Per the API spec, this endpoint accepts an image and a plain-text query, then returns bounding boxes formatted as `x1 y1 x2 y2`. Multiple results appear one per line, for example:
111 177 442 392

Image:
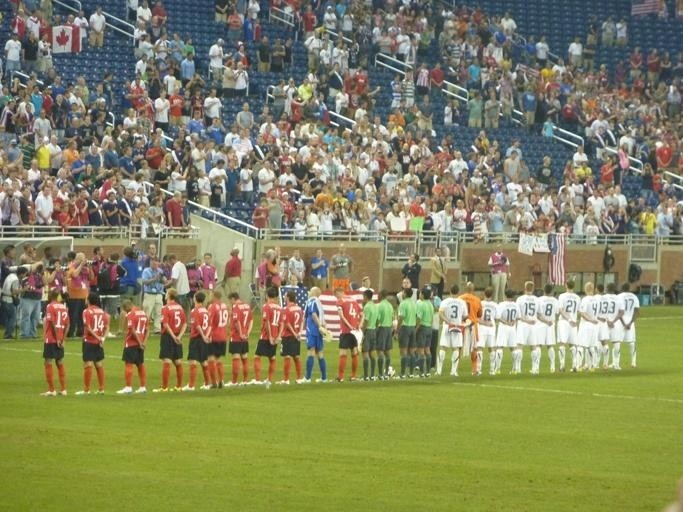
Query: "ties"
439 258 443 273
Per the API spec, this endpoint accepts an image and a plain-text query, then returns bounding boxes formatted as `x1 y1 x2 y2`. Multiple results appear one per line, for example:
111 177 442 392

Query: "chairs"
0 2 679 239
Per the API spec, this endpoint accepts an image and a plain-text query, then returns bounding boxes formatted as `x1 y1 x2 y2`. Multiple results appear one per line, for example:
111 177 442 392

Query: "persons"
0 1 683 340
330 281 640 382
40 287 110 397
115 286 328 395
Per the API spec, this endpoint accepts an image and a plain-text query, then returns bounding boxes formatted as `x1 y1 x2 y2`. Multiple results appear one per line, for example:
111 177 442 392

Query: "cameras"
46 265 56 271
60 265 69 272
195 281 202 287
160 272 166 276
84 260 97 266
185 262 196 269
273 256 288 264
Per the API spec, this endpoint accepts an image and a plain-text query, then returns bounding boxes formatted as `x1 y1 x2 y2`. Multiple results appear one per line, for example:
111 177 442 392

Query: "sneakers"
335 373 431 383
200 382 210 390
94 390 104 395
182 384 195 391
75 390 90 396
58 390 68 396
116 386 132 394
169 385 182 392
211 378 329 389
135 386 147 393
153 385 168 392
41 390 56 397
435 361 636 376
